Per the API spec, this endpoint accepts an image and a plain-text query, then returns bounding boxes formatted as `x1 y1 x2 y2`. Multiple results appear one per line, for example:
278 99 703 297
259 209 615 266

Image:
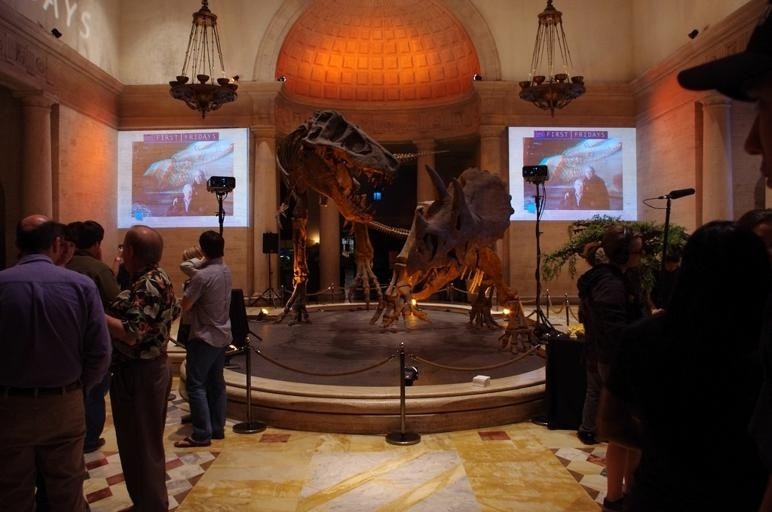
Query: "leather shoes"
175 438 211 447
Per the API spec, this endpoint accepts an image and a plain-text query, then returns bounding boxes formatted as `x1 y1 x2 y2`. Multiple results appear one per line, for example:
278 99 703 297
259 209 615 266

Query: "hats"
677 6 772 103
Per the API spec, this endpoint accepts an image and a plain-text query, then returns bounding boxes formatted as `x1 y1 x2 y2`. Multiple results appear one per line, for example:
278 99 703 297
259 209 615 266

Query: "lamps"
517 0 586 119
168 0 240 122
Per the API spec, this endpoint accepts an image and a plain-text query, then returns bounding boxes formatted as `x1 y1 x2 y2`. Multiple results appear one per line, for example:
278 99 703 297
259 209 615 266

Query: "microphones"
658 188 695 199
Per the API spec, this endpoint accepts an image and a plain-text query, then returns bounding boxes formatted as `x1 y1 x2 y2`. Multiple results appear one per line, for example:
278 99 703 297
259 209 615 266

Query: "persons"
561 178 596 210
572 207 771 510
165 183 209 216
673 5 771 196
0 210 182 512
564 165 611 210
172 229 235 448
177 242 208 426
171 168 222 216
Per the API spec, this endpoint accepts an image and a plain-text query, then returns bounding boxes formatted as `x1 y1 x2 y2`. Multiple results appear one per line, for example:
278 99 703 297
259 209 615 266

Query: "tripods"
249 254 281 307
527 184 556 331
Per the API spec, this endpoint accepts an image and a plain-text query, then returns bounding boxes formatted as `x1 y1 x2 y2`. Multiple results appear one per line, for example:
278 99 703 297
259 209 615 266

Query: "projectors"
206 176 235 192
523 165 550 182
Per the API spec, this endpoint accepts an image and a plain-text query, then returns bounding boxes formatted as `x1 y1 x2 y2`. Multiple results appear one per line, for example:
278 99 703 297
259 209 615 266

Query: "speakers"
263 233 279 252
